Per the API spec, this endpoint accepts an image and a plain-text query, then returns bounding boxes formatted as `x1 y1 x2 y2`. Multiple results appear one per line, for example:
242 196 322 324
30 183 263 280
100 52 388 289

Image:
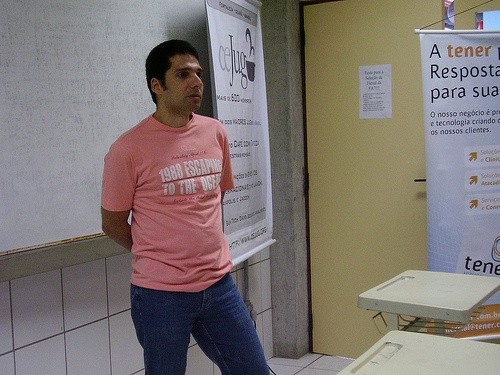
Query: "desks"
334 328 500 375
355 269 500 331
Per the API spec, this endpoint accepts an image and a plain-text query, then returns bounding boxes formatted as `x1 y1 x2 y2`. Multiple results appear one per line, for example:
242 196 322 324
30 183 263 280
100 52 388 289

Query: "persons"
100 40 269 375
